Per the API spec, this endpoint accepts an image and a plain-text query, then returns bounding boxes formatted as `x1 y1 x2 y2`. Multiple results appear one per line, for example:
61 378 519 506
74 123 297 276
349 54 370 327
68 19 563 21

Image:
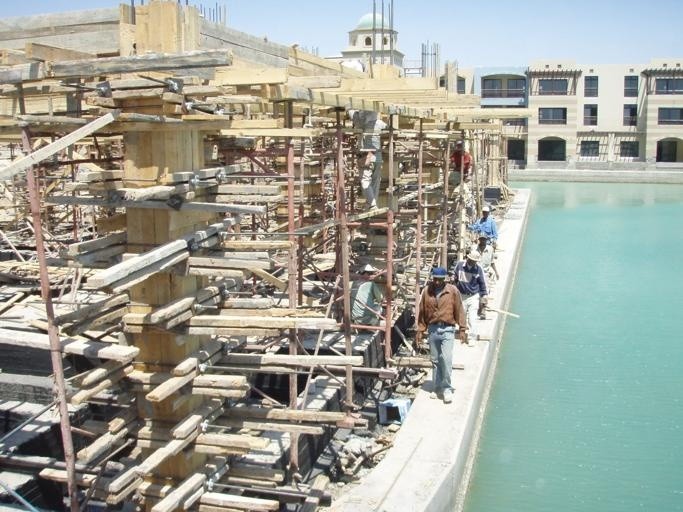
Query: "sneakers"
430 388 441 399
443 388 453 404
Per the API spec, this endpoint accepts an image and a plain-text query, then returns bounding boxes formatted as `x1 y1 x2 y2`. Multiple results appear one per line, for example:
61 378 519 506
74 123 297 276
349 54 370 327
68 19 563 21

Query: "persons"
447 138 471 185
465 205 497 257
449 250 489 347
469 234 500 303
415 266 469 403
351 265 383 332
345 109 388 211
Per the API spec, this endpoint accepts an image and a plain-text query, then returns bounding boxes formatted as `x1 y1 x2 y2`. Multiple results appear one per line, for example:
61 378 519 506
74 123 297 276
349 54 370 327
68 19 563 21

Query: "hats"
479 232 487 239
359 264 377 274
481 206 490 212
466 251 482 262
431 267 447 279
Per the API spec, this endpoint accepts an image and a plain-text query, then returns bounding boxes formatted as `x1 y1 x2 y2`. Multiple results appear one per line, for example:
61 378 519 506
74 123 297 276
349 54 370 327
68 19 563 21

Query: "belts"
432 322 453 327
